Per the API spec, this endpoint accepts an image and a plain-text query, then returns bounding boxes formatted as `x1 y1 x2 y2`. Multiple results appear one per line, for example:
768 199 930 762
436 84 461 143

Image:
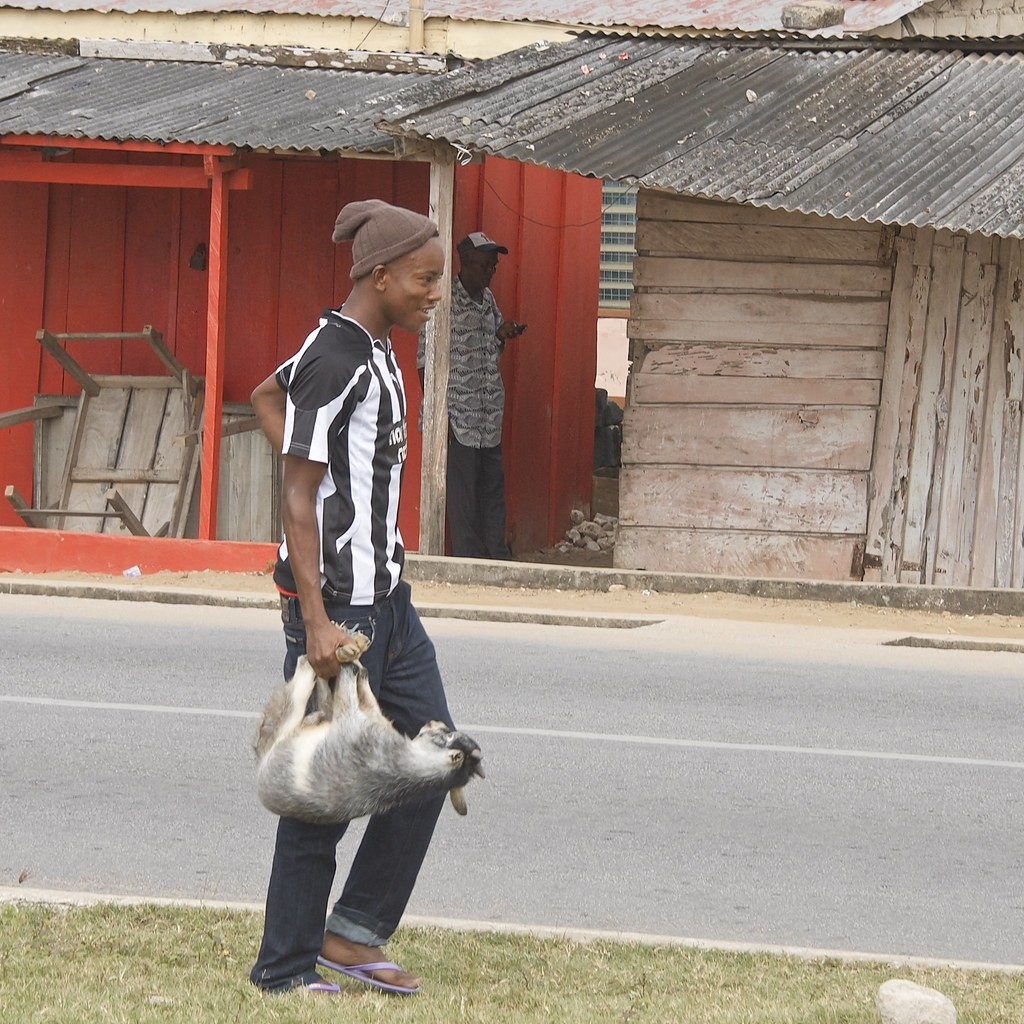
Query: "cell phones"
515 324 525 332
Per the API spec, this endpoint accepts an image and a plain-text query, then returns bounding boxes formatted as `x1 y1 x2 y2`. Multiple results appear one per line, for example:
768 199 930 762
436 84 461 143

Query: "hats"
331 199 438 280
458 231 510 254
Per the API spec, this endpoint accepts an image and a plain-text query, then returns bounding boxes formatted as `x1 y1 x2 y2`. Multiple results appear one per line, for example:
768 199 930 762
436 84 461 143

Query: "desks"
3 325 210 532
0 393 283 544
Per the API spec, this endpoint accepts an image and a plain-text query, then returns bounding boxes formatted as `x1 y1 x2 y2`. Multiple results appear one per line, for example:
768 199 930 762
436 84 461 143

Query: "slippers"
309 978 340 995
317 955 419 993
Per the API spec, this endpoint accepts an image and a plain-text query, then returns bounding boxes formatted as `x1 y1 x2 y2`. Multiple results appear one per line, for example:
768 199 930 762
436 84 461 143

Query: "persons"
416 231 525 563
250 200 455 996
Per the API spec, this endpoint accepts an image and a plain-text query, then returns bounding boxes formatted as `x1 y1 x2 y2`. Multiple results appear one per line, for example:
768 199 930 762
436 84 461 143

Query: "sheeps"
248 619 485 825
